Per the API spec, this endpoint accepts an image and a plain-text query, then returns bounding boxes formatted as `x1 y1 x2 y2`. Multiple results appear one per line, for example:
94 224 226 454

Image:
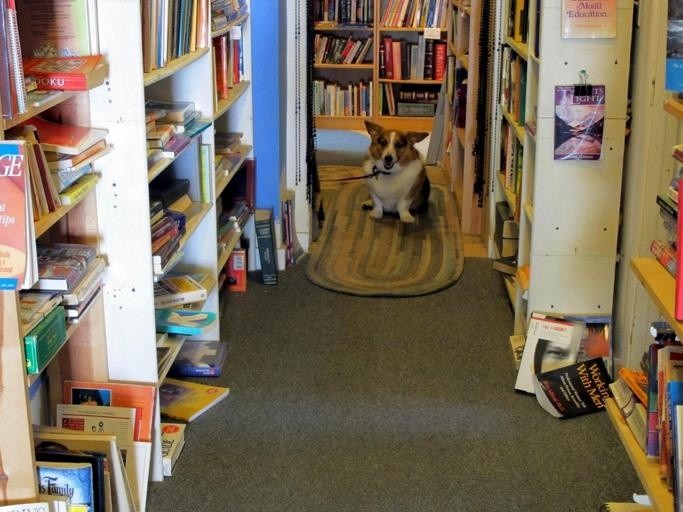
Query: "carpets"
302 178 465 297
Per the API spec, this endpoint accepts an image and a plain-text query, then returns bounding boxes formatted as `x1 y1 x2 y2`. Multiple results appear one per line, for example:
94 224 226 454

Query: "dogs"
361 120 430 224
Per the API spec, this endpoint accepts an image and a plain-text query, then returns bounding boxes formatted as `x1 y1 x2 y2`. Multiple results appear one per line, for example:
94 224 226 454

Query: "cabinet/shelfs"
312 0 376 131
377 1 449 130
0 0 113 512
87 0 217 482
603 2 682 512
488 0 634 374
440 1 483 235
210 1 252 333
